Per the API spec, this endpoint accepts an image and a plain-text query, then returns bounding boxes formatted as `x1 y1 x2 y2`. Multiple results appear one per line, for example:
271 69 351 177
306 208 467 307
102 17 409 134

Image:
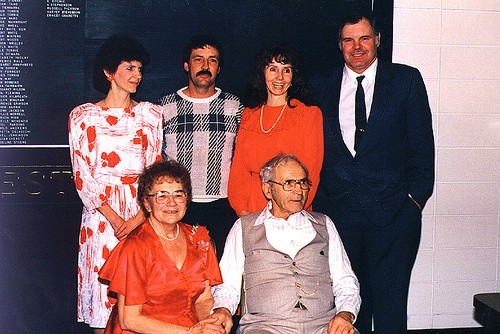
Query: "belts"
250 302 336 315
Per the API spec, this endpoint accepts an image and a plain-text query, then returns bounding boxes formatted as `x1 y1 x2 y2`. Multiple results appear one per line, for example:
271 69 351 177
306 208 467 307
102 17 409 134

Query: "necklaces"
148 217 180 241
260 100 287 133
104 99 132 122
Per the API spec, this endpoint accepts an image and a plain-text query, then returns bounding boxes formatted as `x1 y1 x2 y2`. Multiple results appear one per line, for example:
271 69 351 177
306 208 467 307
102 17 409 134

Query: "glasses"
267 178 312 191
145 190 189 204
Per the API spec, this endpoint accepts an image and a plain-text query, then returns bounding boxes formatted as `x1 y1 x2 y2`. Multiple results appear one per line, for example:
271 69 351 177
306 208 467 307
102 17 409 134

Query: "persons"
68 34 163 334
311 10 435 334
228 44 326 217
156 35 242 263
97 160 227 334
211 154 363 334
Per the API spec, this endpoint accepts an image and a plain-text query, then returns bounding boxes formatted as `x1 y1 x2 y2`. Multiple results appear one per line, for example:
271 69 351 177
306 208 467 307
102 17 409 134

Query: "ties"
354 75 368 152
